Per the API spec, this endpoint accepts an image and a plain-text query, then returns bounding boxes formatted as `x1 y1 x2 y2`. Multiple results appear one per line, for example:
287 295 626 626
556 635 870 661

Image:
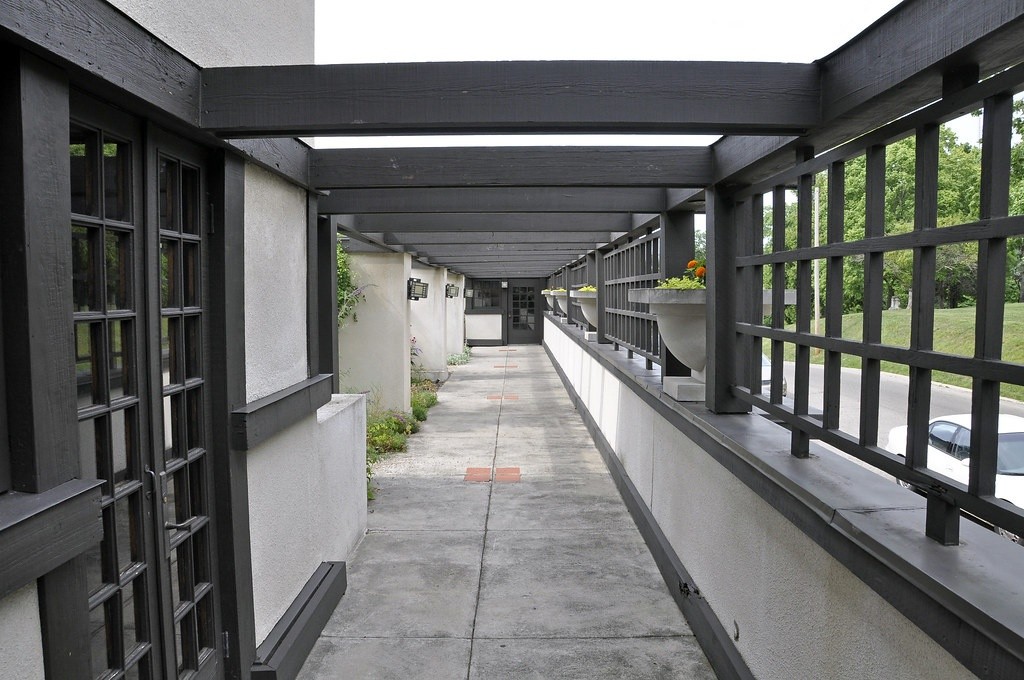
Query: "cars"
761 352 789 398
887 412 1024 544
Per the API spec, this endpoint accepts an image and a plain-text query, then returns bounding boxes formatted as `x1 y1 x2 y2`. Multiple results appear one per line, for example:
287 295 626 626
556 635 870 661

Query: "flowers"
656 259 706 290
578 285 596 292
552 287 566 291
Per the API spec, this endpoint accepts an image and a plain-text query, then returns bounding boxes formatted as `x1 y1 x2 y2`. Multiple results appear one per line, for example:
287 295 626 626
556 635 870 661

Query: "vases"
550 290 567 324
628 289 797 401
569 290 598 341
541 291 553 316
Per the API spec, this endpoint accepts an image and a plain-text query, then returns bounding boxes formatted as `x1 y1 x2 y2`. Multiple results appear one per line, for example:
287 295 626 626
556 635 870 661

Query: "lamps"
464 288 473 298
445 284 459 298
407 278 429 301
